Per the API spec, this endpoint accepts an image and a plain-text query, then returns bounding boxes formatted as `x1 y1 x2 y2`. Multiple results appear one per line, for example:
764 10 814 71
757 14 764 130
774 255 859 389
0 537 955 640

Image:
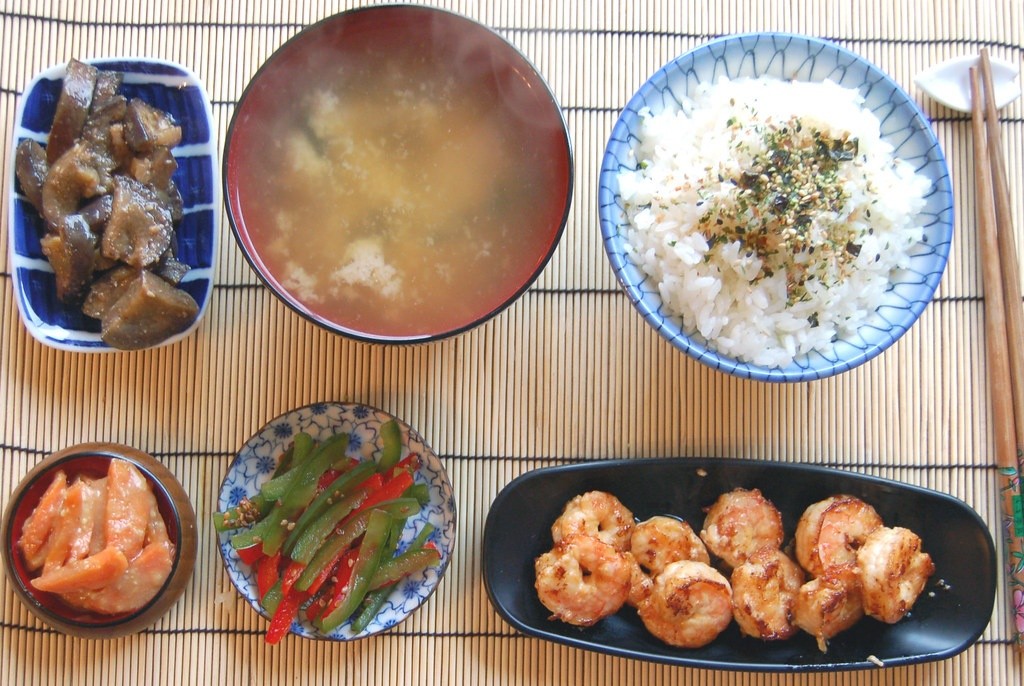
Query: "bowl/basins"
2 442 197 639
220 3 574 344
597 34 953 384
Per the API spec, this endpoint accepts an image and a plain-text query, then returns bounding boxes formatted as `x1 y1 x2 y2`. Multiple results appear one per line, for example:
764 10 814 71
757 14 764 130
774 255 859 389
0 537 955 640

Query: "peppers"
211 419 441 643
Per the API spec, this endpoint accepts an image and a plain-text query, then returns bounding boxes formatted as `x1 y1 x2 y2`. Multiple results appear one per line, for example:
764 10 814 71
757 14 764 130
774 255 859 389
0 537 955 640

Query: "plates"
483 464 996 670
216 402 457 640
12 57 221 351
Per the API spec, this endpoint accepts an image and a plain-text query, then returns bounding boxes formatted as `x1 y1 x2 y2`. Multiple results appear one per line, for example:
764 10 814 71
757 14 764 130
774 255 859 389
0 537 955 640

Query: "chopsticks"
970 47 1023 659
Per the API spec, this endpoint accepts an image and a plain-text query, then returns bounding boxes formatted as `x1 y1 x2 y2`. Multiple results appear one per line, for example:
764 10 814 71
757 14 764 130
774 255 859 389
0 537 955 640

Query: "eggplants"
17 58 200 353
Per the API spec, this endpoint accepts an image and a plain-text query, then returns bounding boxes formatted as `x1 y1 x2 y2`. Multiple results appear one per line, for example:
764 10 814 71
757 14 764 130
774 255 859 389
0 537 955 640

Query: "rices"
620 73 932 369
